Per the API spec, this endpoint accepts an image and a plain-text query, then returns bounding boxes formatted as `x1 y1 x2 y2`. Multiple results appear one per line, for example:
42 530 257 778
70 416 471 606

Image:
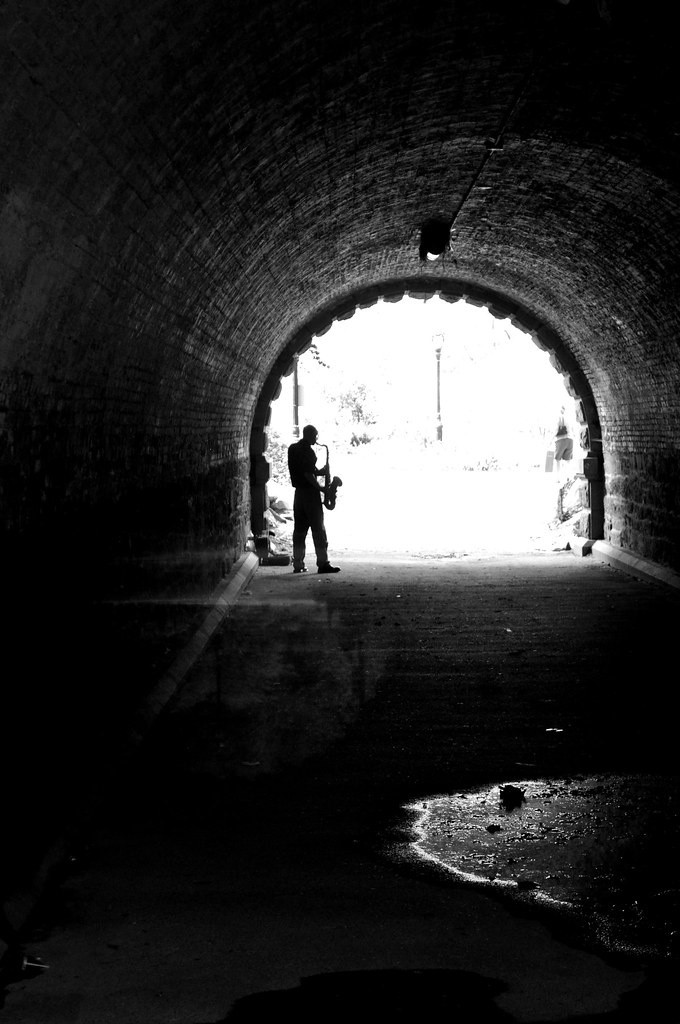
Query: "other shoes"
318 563 341 573
294 566 307 573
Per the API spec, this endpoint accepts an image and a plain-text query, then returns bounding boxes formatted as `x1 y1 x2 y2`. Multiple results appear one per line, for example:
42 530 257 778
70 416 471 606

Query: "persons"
287 425 343 574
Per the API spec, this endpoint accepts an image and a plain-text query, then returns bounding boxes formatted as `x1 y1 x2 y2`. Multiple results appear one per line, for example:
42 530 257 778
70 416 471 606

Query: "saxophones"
316 442 342 510
